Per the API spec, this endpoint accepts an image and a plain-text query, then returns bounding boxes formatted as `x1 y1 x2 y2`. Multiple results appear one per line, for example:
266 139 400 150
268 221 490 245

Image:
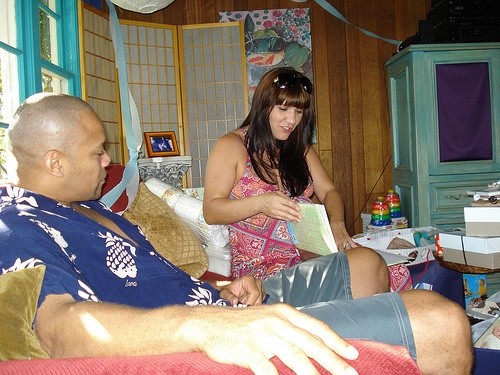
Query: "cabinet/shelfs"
383 42 500 297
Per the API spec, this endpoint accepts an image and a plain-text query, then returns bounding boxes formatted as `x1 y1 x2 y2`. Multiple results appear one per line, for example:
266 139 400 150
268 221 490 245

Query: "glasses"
272 73 312 94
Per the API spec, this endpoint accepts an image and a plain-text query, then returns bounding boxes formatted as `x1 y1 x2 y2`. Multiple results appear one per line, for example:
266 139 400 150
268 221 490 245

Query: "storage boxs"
463 206 500 237
205 243 232 277
353 226 466 311
439 231 500 269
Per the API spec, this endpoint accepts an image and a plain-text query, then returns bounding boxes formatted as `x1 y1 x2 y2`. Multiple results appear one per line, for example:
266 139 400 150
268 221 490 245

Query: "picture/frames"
144 131 181 158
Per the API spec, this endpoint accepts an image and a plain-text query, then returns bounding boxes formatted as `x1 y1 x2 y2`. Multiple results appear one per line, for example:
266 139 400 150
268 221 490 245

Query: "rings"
346 244 350 246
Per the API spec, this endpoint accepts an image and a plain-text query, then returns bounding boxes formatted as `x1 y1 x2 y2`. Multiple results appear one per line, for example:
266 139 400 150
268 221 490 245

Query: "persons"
150 136 172 152
478 279 487 300
203 68 412 294
0 92 473 375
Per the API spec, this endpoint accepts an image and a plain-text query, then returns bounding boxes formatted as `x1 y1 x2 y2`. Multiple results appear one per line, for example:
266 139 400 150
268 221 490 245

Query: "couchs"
0 162 425 375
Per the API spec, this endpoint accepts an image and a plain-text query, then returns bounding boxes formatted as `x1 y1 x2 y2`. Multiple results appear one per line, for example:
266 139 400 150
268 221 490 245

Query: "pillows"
123 181 209 278
0 264 51 362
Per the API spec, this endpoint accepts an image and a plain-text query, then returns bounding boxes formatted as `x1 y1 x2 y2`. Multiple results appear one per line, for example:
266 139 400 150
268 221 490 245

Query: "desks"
436 255 500 274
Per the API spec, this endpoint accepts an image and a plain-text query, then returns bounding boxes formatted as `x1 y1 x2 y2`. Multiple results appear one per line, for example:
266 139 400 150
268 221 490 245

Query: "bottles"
371 196 392 226
386 189 403 218
